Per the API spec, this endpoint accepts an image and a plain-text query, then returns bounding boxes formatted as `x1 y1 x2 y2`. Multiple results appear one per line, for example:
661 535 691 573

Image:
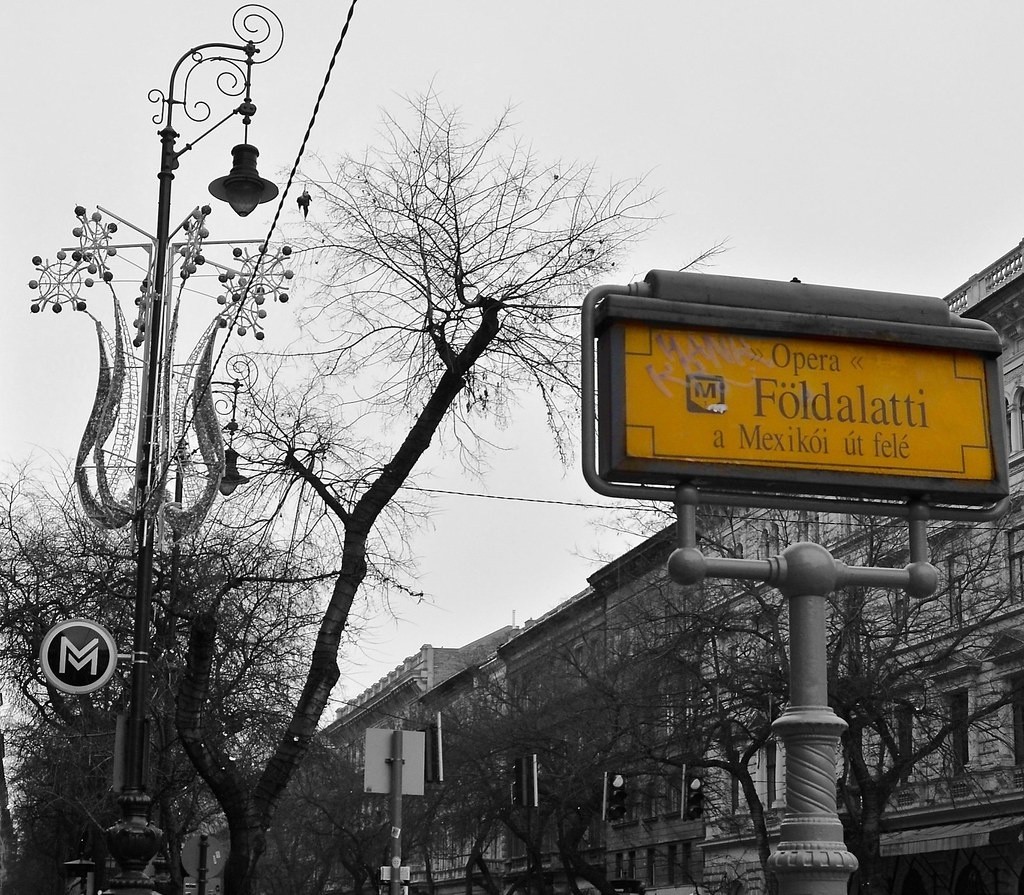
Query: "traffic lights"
607 772 628 820
686 775 705 820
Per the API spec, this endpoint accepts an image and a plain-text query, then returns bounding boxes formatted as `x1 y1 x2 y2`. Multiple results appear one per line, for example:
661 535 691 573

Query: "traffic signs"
582 270 1008 521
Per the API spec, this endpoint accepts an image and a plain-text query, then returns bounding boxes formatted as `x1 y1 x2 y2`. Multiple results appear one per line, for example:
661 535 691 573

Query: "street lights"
30 2 293 893
